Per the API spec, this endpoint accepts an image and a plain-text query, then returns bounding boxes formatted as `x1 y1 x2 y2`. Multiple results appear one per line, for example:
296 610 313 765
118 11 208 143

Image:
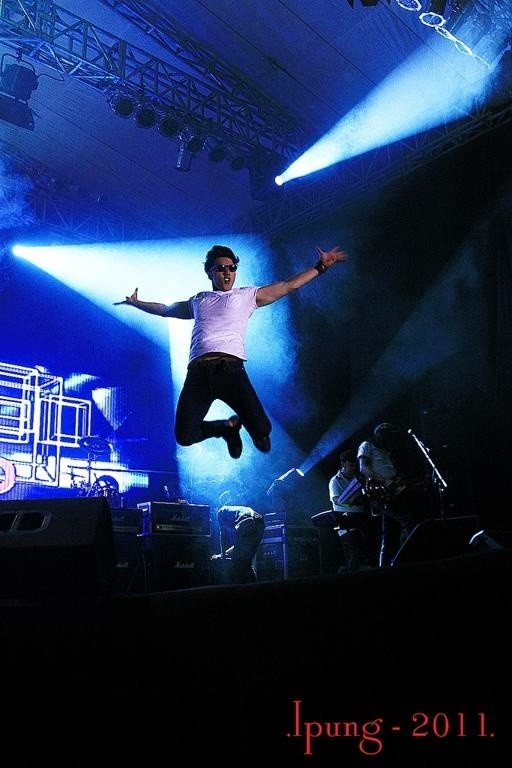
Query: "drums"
93 486 121 505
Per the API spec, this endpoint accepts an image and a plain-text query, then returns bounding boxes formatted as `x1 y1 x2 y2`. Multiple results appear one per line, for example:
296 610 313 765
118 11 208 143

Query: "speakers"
0 497 116 588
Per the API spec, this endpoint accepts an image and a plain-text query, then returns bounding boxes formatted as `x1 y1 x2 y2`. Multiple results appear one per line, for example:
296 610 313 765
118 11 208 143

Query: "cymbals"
77 435 113 457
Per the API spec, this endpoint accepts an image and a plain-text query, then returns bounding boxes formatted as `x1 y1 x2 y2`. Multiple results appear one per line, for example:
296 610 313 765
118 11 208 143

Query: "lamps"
179 120 205 156
174 145 196 175
205 135 230 164
0 47 66 133
156 114 179 141
132 103 160 131
253 177 285 204
108 90 136 119
225 145 248 175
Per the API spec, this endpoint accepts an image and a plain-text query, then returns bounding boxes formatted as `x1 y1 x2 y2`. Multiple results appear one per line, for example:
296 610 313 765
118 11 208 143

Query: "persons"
112 245 350 460
208 505 266 585
357 421 422 548
328 450 370 567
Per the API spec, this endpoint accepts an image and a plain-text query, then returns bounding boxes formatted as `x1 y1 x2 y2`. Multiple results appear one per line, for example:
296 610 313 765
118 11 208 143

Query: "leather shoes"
252 436 272 453
226 415 242 460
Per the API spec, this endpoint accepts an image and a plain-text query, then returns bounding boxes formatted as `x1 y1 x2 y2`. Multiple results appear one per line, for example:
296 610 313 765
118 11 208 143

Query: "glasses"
210 263 239 274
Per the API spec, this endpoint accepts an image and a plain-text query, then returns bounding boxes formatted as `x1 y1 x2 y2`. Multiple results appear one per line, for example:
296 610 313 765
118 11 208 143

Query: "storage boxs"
255 510 321 580
136 499 211 536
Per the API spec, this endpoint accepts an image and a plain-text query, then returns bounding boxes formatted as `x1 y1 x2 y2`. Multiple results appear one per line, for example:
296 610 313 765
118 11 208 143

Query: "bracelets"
312 260 328 275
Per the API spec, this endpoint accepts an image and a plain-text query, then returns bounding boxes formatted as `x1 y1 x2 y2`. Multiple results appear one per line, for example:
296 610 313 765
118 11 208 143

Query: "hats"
373 423 395 435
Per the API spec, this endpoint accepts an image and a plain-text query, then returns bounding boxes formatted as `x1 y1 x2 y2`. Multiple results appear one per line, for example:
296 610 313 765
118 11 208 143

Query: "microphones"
406 429 431 452
